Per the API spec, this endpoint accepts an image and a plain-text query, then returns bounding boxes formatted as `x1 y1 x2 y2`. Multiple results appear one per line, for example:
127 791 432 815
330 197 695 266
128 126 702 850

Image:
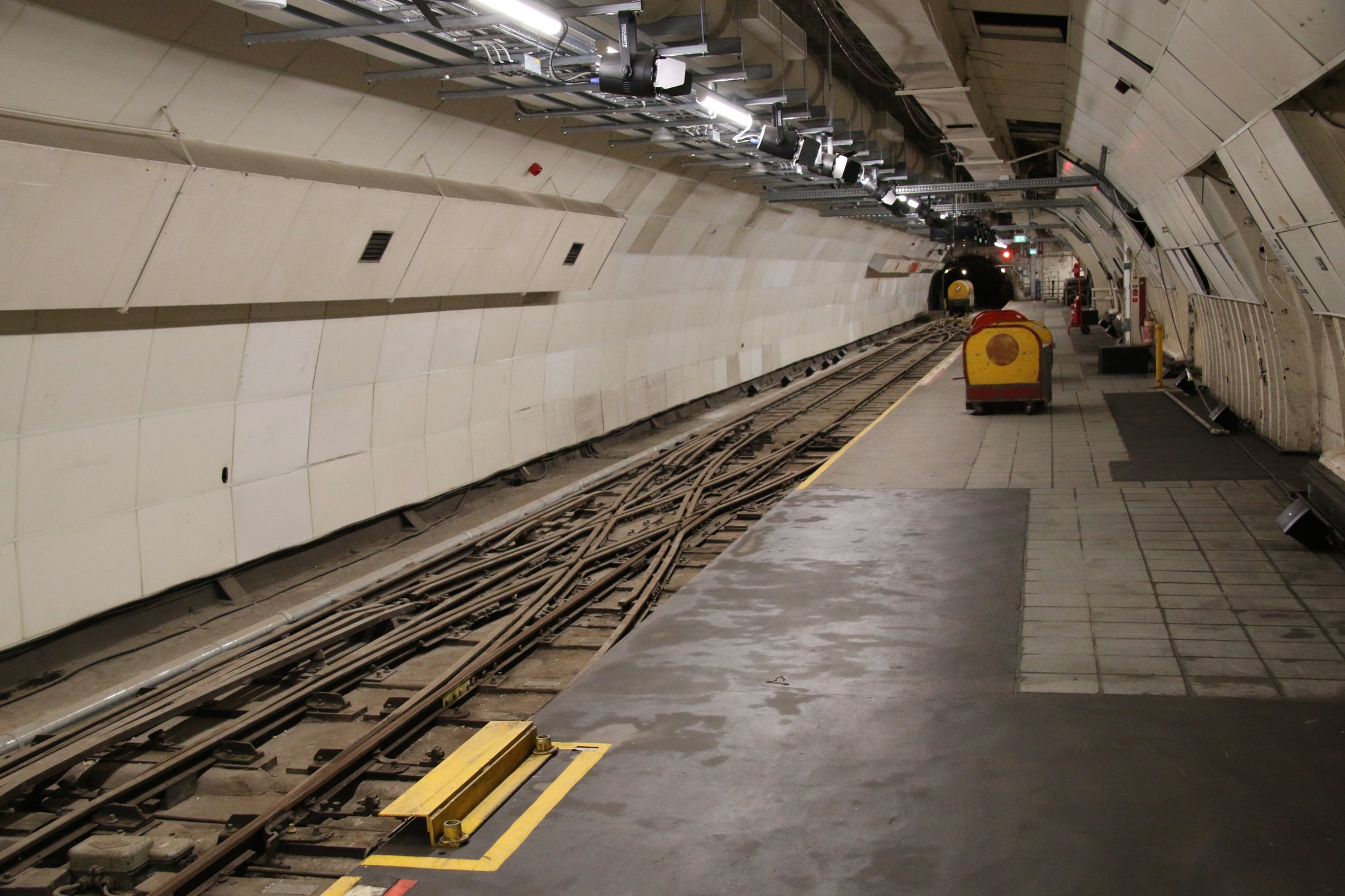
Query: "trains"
947 279 975 316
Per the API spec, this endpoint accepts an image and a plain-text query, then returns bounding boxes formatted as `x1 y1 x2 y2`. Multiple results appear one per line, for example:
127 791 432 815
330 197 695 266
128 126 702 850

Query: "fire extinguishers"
1071 299 1080 325
1072 263 1079 277
1139 309 1156 343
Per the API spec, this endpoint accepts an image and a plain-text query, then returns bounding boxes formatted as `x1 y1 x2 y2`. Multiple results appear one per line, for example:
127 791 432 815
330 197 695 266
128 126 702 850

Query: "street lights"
940 265 966 310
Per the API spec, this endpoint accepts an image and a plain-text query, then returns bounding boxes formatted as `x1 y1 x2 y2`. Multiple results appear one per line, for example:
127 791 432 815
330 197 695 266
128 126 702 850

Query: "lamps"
597 10 693 99
1172 370 1197 396
756 102 999 250
1098 318 1109 329
1208 401 1239 432
693 91 752 127
465 0 563 36
1104 324 1119 338
1273 499 1334 553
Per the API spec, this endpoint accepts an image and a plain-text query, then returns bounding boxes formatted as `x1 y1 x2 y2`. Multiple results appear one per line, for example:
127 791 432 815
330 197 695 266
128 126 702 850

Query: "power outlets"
1314 256 1328 272
